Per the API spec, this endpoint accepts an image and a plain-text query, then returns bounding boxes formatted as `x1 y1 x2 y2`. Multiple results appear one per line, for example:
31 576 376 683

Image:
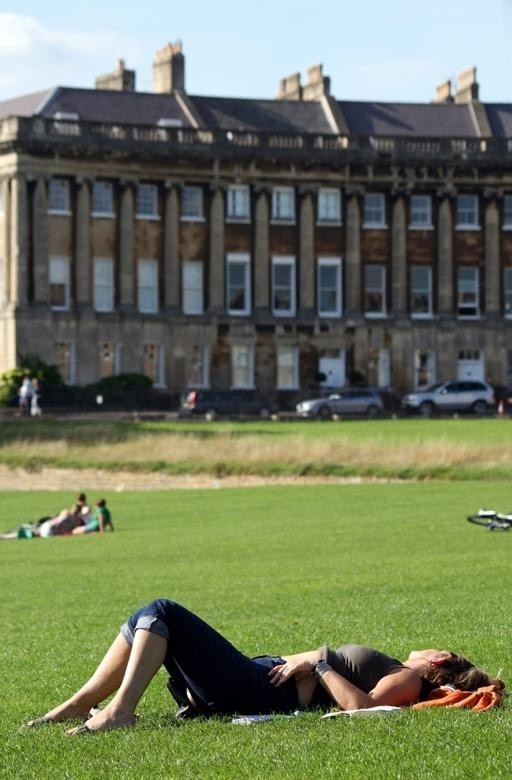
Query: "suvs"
399 378 498 416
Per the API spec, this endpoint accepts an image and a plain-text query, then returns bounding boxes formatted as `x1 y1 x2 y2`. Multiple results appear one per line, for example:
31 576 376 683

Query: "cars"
294 387 387 418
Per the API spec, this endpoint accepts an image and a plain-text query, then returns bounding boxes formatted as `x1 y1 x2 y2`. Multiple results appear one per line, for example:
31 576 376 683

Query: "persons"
15 369 32 418
75 493 92 526
27 372 43 417
30 502 83 540
0 516 52 541
68 498 116 535
24 597 490 739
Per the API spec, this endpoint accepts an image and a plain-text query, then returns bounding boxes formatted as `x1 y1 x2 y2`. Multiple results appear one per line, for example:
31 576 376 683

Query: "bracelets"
311 659 332 680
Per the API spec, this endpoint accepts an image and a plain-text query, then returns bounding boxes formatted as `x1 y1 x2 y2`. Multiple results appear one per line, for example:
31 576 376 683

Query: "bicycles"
465 507 512 533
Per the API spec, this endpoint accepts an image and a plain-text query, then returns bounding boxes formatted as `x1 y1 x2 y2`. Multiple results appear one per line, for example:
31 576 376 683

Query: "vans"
184 388 284 422
